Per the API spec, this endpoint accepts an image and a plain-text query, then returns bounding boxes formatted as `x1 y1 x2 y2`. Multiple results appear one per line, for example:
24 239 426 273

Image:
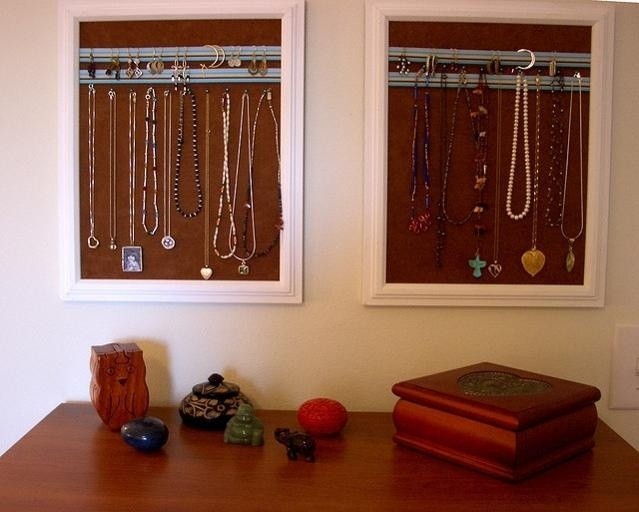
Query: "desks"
0 402 638 512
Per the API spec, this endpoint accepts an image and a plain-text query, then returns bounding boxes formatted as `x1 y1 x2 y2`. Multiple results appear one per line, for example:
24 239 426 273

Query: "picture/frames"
361 0 616 308
54 1 306 303
